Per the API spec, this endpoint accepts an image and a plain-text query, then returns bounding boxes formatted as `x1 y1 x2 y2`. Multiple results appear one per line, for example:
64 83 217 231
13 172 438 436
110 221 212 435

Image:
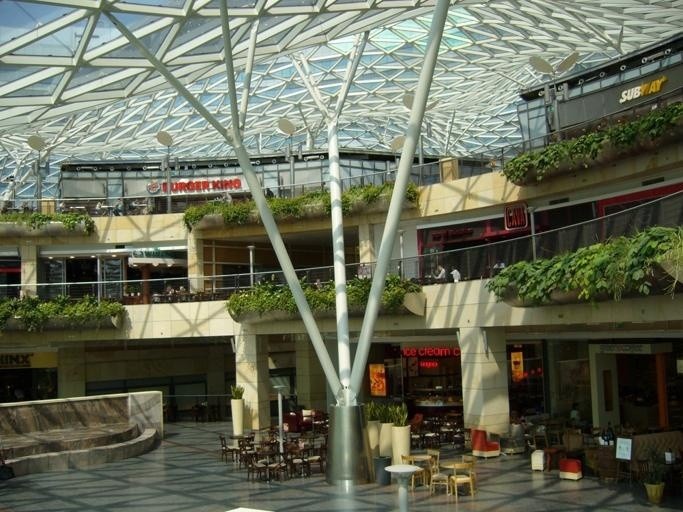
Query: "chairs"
218 427 327 484
401 450 476 500
636 459 648 493
527 416 634 485
405 410 464 449
470 430 499 461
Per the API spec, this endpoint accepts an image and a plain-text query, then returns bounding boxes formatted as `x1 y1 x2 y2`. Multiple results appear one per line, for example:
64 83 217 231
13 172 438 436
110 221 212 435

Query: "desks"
489 432 526 456
653 456 681 496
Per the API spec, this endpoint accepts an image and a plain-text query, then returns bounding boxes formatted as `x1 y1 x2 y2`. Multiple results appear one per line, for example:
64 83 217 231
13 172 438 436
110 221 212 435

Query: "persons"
570 403 579 429
433 265 447 282
59 199 149 216
493 260 505 269
450 266 461 282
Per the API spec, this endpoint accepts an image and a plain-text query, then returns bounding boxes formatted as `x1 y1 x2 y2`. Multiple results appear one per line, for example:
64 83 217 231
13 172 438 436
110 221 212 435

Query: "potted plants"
229 384 245 460
363 400 410 467
640 446 668 504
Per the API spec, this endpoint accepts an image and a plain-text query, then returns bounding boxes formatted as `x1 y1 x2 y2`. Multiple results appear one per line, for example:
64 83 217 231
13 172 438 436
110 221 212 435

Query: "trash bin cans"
41 198 56 217
374 456 391 485
438 157 459 182
